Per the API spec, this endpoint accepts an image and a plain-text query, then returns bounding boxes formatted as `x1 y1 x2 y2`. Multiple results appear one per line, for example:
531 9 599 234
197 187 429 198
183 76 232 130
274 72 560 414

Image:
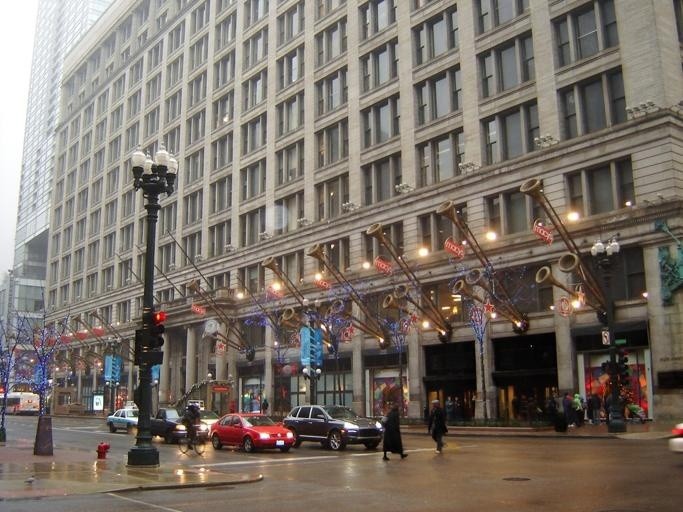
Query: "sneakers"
435 450 442 454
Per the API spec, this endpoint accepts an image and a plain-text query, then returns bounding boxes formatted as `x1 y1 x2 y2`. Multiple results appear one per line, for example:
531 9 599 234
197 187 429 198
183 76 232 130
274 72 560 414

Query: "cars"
668 422 683 454
148 406 209 443
195 408 222 438
104 408 155 434
208 412 294 453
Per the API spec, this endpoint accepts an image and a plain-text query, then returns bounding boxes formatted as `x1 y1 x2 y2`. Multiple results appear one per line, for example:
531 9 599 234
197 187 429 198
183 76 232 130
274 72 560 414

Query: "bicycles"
177 435 207 456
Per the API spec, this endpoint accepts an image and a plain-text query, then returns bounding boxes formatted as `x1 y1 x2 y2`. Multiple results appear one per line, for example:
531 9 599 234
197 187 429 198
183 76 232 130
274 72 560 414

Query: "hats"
431 400 439 404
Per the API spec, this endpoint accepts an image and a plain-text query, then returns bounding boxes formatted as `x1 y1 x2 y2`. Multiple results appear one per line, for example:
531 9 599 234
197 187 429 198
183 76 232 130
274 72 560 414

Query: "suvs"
281 403 383 453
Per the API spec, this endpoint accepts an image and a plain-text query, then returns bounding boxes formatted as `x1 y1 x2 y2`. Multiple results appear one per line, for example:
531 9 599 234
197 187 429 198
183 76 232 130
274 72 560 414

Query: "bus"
0 390 40 416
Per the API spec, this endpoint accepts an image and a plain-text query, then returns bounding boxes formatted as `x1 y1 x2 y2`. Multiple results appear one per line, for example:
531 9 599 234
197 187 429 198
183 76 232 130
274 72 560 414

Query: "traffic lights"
147 310 166 350
617 352 630 386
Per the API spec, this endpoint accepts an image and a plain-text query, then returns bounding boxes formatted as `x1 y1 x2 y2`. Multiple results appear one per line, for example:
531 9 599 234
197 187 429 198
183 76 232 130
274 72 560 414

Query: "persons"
508 386 613 429
426 398 448 454
445 396 454 421
453 397 461 420
181 403 200 450
230 398 268 420
382 403 408 460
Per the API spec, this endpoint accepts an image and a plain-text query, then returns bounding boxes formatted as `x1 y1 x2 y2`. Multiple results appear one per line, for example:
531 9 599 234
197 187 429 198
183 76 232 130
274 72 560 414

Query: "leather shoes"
401 454 407 458
383 457 389 460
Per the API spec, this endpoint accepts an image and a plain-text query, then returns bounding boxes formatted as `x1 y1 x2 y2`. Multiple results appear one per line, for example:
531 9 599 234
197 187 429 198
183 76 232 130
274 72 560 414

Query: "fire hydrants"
94 440 110 459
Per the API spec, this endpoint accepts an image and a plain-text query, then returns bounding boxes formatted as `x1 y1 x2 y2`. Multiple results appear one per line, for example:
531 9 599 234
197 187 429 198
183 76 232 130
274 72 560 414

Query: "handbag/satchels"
381 416 388 428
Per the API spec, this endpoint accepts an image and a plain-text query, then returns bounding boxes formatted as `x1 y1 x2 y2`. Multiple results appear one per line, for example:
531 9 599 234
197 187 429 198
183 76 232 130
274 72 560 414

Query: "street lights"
587 231 628 433
126 141 181 467
106 381 120 412
300 367 322 404
